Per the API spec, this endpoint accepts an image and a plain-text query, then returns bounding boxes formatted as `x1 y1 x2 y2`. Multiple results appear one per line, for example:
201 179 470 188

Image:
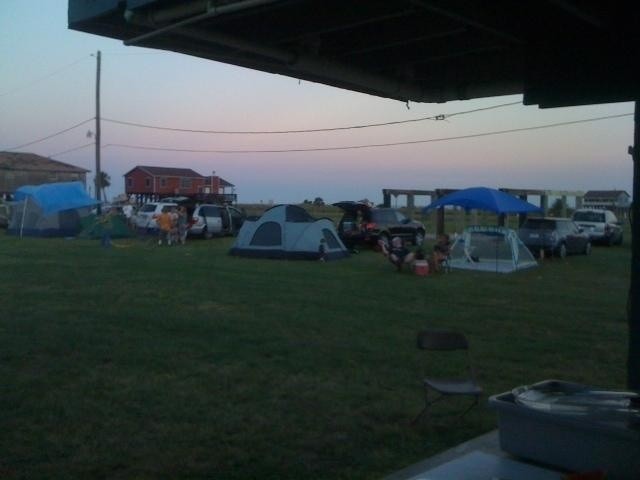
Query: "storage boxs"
413 259 430 277
342 222 355 231
488 379 640 480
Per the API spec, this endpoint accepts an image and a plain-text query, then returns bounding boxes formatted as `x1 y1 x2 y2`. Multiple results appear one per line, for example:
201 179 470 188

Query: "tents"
440 225 538 274
226 202 351 261
7 180 100 238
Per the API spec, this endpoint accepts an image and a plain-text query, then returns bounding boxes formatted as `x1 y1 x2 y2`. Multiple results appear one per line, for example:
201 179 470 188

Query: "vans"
332 199 425 248
134 197 246 240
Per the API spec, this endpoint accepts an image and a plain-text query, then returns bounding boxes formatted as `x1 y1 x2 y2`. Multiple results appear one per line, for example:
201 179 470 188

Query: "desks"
379 430 570 480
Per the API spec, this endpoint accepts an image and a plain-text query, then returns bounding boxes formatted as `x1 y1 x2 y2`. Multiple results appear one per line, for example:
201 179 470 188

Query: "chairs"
412 330 484 438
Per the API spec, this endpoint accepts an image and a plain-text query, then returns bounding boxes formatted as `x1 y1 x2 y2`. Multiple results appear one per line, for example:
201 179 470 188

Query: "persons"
122 202 133 221
376 236 424 271
156 206 188 246
318 238 328 262
431 233 450 273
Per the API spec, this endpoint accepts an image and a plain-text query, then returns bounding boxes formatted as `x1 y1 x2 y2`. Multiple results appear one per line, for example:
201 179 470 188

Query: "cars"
572 208 623 247
519 217 591 257
